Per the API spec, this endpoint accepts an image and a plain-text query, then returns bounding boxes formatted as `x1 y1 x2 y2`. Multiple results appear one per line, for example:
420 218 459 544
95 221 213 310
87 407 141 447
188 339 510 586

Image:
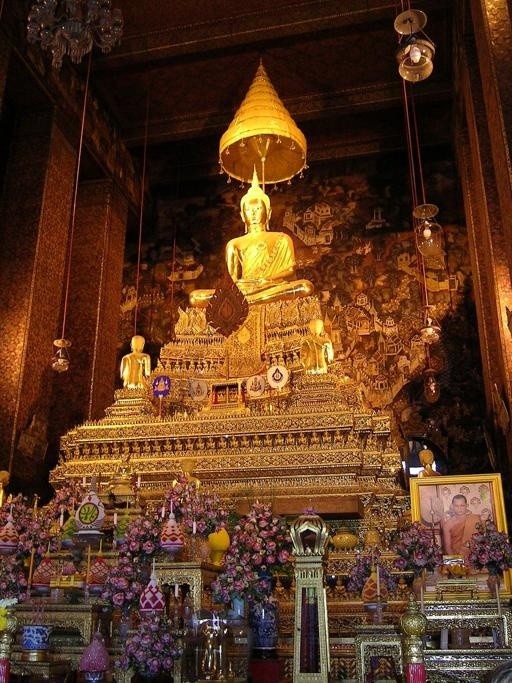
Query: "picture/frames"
407 472 511 597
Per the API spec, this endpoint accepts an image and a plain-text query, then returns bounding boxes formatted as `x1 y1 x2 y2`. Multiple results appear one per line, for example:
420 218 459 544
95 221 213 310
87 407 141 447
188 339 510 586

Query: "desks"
408 597 512 682
351 621 409 682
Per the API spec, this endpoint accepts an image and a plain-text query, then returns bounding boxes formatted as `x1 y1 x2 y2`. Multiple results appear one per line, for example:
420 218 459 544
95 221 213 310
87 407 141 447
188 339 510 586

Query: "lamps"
26 0 126 70
409 85 446 256
47 52 93 375
390 0 435 81
406 128 443 347
409 170 441 404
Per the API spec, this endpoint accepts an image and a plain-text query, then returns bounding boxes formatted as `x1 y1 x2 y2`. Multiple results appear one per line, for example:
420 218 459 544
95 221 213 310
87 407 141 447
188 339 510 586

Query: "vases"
412 577 426 599
486 575 501 598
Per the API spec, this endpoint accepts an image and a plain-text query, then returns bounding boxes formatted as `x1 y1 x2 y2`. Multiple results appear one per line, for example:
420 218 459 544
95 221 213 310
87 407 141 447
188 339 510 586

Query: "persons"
443 494 480 555
419 451 440 477
173 460 201 488
300 319 334 375
0 470 11 508
188 163 312 306
120 335 152 389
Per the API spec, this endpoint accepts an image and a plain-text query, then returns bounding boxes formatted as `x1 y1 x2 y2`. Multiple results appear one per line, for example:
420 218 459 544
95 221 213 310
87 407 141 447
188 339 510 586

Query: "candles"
376 565 381 595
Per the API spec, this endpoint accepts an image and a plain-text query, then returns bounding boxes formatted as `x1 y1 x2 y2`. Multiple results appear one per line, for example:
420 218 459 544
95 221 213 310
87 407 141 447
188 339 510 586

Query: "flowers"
347 545 397 596
392 520 443 583
1 472 296 683
461 515 512 574
